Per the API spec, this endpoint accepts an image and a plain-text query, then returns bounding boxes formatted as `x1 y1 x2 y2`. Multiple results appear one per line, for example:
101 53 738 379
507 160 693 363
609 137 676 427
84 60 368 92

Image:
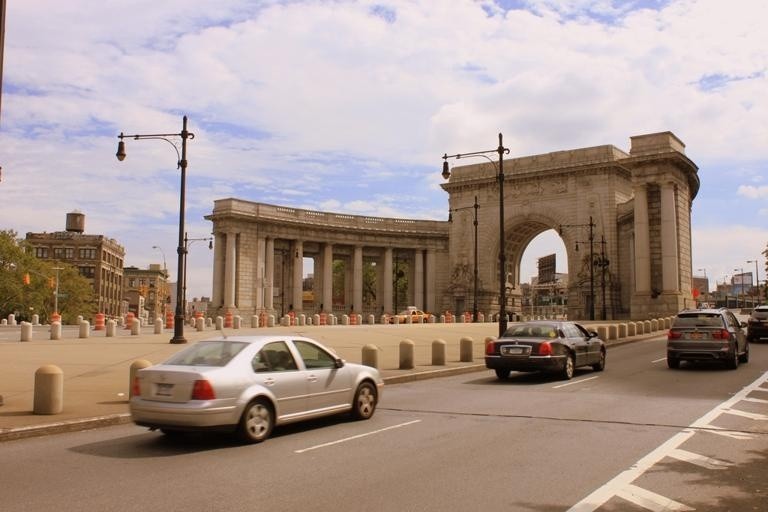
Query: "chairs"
260 349 278 365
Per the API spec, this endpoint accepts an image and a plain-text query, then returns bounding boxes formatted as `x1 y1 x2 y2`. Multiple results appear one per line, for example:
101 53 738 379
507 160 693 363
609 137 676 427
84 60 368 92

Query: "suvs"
666 307 750 371
747 304 767 341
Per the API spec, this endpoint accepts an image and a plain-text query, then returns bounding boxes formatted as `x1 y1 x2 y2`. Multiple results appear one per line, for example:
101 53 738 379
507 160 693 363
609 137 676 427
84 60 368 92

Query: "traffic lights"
23 273 30 285
47 277 54 288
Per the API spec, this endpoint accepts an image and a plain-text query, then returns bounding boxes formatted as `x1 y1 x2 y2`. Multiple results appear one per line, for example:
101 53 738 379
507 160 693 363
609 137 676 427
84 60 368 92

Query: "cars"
386 306 430 323
127 334 385 446
485 321 607 383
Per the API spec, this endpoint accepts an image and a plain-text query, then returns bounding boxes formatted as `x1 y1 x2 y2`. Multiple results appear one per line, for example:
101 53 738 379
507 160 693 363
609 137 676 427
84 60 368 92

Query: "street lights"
280 245 300 319
152 246 167 324
575 235 608 321
183 232 213 325
558 216 596 320
441 132 509 339
724 259 760 311
447 196 480 322
698 268 708 303
115 116 196 344
